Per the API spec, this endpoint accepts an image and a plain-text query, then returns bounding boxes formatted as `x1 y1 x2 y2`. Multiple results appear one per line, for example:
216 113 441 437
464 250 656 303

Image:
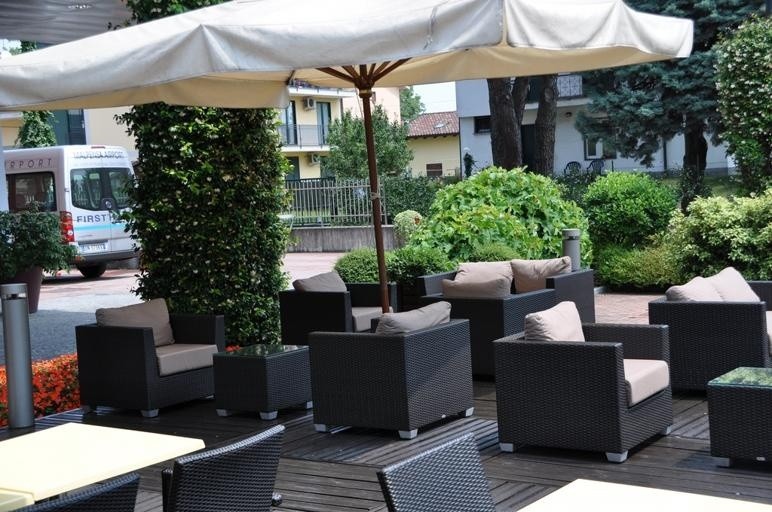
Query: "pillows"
703 267 761 301
96 299 175 348
523 299 585 342
666 277 724 302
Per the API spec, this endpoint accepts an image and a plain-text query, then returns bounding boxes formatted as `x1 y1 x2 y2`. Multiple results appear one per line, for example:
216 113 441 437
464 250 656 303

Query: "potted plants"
0 198 80 314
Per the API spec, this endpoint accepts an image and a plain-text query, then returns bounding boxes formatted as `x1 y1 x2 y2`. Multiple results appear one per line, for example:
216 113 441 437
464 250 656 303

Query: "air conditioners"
301 97 316 111
306 152 320 166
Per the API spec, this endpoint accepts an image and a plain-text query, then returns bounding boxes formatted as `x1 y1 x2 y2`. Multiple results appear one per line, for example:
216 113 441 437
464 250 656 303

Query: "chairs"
377 435 493 510
563 161 581 176
160 425 285 510
12 472 142 510
587 159 605 175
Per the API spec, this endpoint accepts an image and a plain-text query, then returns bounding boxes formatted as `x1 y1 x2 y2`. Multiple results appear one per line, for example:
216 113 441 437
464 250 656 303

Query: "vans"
0 143 146 283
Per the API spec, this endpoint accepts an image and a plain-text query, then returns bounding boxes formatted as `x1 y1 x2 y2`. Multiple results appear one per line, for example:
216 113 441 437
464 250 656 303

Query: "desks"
518 480 772 510
2 422 205 501
707 367 772 466
212 343 312 421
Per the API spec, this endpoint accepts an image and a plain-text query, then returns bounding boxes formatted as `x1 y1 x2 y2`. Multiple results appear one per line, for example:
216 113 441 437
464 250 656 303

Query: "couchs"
493 322 674 462
74 297 227 421
648 281 772 400
278 256 595 440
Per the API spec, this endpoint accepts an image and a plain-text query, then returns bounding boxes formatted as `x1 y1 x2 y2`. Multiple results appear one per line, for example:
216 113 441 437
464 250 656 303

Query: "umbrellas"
1 0 694 317
